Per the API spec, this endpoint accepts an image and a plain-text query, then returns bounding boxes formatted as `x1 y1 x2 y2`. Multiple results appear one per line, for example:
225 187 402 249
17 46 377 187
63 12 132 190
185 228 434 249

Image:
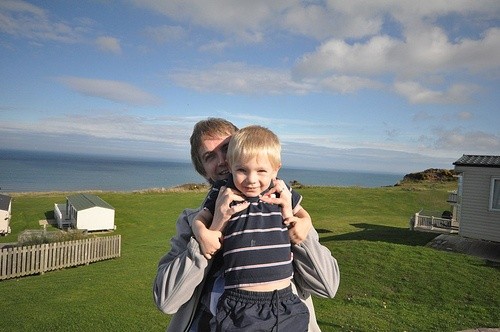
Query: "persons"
153 119 340 332
190 125 313 331
